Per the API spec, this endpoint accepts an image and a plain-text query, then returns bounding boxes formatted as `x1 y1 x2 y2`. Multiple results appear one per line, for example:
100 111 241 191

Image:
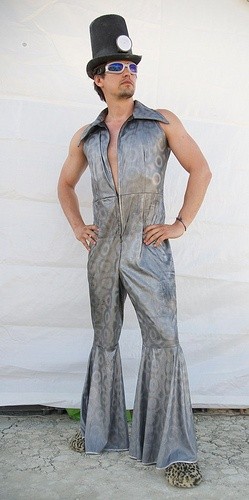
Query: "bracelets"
174 216 186 233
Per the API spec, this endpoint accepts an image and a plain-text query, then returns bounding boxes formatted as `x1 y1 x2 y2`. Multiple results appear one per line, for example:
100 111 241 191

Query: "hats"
86 13 142 80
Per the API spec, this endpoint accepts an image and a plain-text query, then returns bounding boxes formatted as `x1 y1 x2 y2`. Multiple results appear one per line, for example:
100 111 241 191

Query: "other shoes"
164 461 202 489
70 432 86 454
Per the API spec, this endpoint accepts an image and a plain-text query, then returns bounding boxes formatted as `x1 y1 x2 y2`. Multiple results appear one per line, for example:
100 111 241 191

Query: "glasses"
96 62 138 74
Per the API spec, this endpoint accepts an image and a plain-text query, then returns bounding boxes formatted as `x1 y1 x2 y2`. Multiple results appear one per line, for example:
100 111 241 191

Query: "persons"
55 12 213 490
